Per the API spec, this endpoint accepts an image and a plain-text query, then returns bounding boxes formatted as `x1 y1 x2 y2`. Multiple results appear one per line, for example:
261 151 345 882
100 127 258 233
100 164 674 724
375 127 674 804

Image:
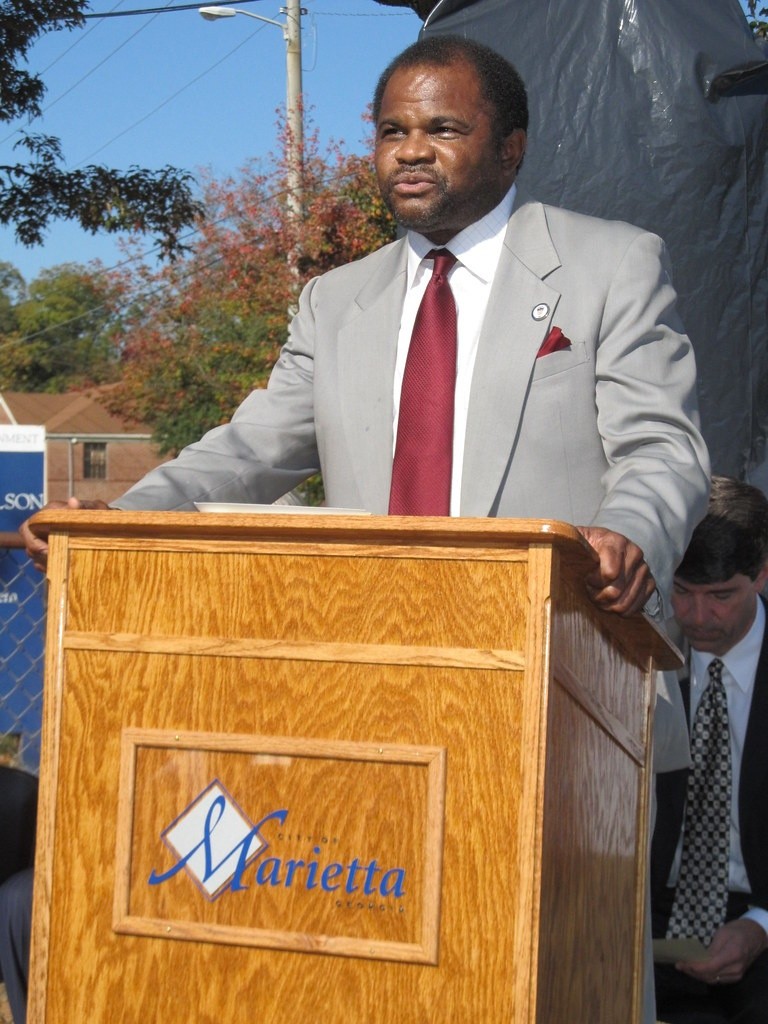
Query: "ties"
663 657 730 950
387 249 457 516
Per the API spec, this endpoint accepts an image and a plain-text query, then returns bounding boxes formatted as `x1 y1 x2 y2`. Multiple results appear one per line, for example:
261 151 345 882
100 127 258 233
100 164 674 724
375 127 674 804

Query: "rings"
715 975 721 984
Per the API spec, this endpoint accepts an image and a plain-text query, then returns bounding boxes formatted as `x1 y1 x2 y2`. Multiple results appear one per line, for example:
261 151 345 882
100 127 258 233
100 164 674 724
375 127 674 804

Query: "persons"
651 474 768 1024
18 33 713 621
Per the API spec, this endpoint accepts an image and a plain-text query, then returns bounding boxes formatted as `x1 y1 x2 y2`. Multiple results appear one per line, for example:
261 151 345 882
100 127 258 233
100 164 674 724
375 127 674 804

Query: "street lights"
198 5 304 327
